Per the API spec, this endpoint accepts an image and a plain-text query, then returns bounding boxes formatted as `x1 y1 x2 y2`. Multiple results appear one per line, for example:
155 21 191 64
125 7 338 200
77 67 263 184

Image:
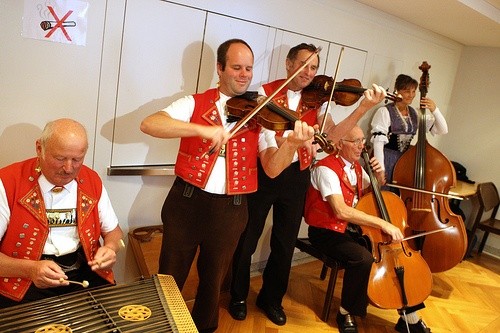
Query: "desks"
448 179 479 229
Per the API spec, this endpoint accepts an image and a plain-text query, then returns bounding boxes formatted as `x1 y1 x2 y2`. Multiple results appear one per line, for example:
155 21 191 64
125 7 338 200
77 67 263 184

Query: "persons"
304 126 433 333
369 74 448 196
221 43 387 326
140 38 319 333
0 118 124 310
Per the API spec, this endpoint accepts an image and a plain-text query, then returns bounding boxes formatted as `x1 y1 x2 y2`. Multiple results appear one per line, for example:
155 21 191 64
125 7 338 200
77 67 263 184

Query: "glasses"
341 137 366 146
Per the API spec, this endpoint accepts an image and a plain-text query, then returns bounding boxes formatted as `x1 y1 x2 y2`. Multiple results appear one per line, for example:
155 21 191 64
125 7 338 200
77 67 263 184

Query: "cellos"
392 61 468 273
354 142 433 333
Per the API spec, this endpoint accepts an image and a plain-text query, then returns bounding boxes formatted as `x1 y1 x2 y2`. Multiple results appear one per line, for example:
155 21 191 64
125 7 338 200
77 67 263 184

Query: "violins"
301 73 403 109
226 91 336 155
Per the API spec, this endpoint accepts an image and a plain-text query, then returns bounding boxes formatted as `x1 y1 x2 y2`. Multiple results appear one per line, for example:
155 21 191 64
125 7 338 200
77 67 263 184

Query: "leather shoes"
256 296 286 326
228 298 247 320
394 317 432 333
336 311 357 333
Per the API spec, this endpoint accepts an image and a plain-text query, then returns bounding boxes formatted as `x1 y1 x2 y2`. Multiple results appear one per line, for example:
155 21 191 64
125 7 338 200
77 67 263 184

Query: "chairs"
464 181 500 257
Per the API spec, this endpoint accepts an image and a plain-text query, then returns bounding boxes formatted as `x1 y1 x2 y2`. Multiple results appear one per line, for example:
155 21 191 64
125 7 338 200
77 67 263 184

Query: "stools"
295 239 345 321
